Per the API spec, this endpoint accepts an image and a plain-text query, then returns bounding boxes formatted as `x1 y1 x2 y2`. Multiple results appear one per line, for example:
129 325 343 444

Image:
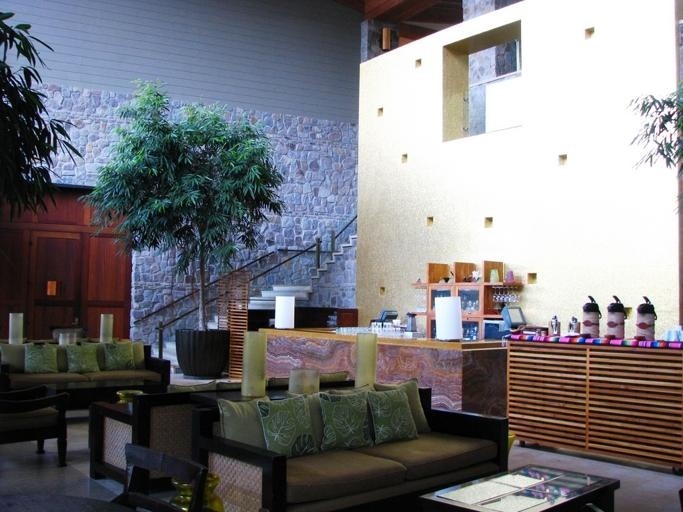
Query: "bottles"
580 295 657 341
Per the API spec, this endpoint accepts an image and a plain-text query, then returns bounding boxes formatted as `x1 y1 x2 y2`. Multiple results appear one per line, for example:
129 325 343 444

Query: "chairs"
0 385 69 469
109 441 209 511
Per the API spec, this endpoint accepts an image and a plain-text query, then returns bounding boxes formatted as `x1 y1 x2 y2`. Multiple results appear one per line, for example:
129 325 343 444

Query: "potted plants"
74 77 288 378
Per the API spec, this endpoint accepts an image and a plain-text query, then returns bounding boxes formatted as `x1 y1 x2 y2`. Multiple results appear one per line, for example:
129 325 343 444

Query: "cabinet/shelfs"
411 260 524 339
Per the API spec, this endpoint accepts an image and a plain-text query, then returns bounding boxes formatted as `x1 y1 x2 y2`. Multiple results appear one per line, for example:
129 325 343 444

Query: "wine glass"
491 286 521 304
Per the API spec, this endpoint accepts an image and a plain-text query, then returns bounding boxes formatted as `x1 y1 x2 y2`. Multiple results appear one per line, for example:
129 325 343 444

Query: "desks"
1 492 136 512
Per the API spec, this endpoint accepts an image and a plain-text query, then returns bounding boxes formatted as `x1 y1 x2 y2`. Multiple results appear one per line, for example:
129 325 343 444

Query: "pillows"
23 340 136 374
256 388 421 459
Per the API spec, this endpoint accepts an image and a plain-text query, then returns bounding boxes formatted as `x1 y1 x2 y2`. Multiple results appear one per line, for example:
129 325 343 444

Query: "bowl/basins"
170 472 220 497
116 389 143 399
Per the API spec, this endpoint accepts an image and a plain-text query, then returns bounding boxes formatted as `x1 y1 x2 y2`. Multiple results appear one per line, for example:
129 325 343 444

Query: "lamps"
356 334 377 389
241 329 269 397
99 313 114 344
274 294 295 330
433 296 464 341
8 311 23 345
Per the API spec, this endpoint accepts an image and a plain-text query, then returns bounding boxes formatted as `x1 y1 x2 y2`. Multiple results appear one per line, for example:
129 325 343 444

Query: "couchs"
0 339 171 410
196 388 509 512
87 371 355 495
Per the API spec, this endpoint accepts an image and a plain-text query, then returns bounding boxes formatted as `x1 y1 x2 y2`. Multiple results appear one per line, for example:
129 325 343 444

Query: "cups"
334 320 405 339
